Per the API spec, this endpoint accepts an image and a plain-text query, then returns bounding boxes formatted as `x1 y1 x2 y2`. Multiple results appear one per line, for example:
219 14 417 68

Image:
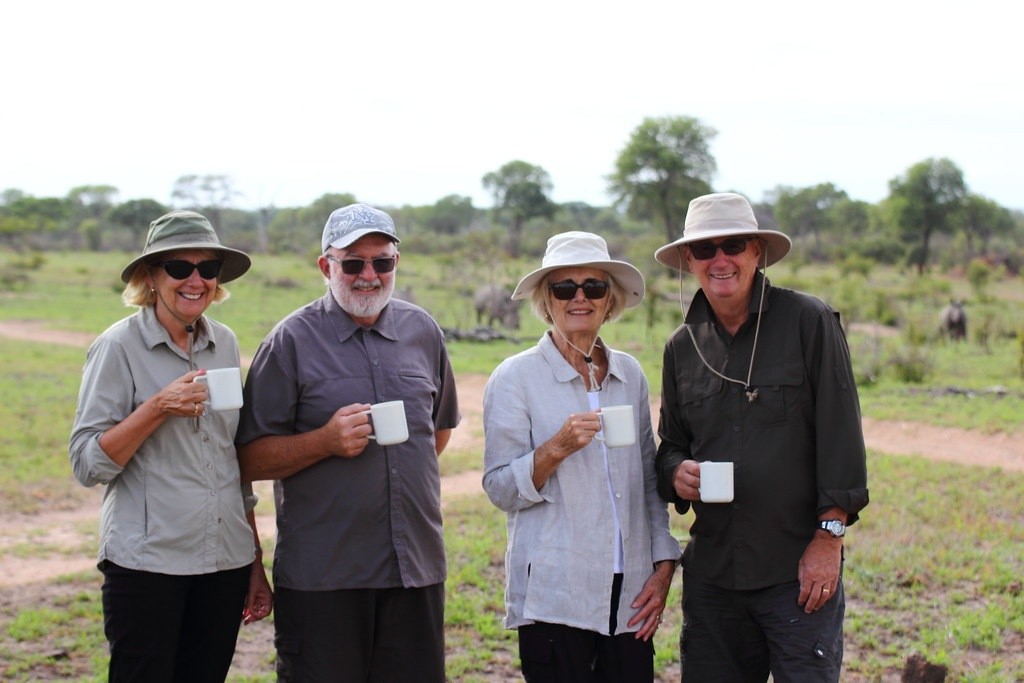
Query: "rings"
823 589 830 592
656 615 663 624
193 404 200 415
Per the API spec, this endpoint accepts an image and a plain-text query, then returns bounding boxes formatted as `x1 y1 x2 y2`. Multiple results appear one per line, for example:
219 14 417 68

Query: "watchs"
816 518 848 538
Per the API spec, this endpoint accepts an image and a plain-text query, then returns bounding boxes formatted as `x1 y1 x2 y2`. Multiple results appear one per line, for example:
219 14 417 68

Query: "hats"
511 231 645 308
653 193 792 274
321 203 401 253
120 210 252 285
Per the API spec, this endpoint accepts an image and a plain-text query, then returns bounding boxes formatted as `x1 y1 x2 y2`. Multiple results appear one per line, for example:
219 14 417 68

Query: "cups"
698 462 734 503
594 405 637 447
360 400 409 445
193 368 244 411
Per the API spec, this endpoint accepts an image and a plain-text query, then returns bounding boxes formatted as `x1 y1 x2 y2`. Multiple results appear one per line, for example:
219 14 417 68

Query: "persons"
238 204 464 683
654 192 871 682
66 211 273 683
480 229 685 683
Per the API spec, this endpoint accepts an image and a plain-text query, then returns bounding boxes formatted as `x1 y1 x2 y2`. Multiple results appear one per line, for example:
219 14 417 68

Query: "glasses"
328 252 397 275
689 236 758 260
155 260 223 280
548 280 609 300
